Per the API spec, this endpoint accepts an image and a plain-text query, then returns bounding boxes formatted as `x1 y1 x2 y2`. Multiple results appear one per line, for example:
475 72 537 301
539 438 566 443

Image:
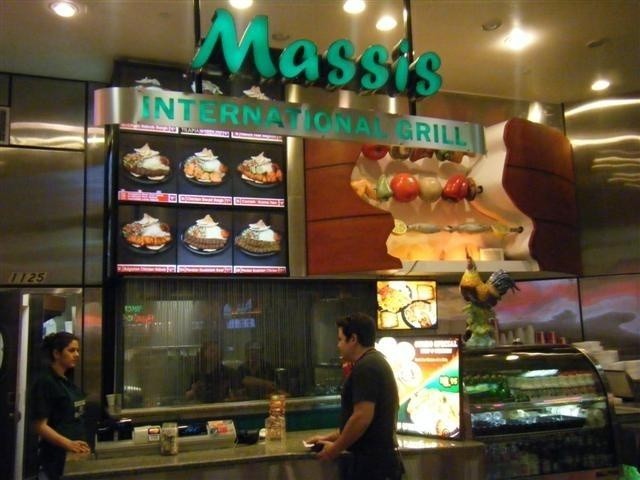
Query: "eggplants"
391 173 419 202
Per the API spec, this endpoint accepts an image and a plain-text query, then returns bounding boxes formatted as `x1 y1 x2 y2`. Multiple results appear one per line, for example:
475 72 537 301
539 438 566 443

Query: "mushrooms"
465 177 483 201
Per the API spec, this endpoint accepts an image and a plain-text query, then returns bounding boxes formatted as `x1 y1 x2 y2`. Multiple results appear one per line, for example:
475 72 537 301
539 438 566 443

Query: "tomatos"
419 177 441 201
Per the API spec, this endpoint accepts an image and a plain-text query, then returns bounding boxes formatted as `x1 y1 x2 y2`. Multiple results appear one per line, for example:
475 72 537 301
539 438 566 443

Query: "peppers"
412 147 432 161
435 150 452 161
376 174 393 202
442 176 469 203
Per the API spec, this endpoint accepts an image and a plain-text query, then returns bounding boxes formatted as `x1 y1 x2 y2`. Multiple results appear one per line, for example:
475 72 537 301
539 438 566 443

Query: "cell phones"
307 441 324 452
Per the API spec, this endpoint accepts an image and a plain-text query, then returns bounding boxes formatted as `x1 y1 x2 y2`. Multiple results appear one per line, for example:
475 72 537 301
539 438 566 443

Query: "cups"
498 323 567 347
267 392 286 414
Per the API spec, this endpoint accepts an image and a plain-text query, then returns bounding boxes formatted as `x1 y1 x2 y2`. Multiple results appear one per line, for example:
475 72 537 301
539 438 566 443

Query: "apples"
361 143 389 161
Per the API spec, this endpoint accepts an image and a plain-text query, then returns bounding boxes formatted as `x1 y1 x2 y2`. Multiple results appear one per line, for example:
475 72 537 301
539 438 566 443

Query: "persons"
302 313 404 480
29 331 93 479
185 339 239 402
234 337 289 399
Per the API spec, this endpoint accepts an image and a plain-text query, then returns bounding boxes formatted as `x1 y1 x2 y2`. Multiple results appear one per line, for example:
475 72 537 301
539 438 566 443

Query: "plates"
122 227 174 255
376 283 413 314
179 223 232 256
236 229 284 258
180 155 230 186
238 174 282 188
121 155 177 186
401 299 437 329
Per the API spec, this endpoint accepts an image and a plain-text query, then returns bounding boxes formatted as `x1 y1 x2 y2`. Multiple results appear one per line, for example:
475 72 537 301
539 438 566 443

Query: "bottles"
265 408 287 455
460 371 597 405
543 430 613 475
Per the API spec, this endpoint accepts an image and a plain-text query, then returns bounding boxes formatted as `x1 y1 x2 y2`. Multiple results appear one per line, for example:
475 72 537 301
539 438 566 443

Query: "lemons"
392 218 407 234
491 224 508 235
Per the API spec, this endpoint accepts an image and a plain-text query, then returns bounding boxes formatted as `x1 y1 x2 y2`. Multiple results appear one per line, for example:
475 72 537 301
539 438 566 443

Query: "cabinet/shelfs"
461 344 620 479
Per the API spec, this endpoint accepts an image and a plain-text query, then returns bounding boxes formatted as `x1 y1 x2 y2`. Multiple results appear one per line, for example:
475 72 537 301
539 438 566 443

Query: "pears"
390 146 410 160
